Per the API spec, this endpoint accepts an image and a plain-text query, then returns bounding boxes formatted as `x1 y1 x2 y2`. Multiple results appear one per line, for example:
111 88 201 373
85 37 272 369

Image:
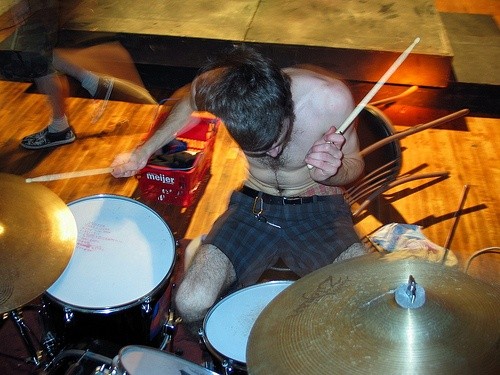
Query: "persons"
8 1 113 150
111 43 367 321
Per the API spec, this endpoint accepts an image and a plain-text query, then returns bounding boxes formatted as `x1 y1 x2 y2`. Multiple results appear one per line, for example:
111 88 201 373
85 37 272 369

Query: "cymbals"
0 172 77 315
246 254 500 375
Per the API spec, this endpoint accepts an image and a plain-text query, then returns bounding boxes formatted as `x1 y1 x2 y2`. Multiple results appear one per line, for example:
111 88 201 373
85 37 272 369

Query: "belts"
240 185 346 205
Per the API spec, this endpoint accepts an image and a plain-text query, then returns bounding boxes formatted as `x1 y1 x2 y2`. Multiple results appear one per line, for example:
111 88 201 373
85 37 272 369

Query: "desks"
58 0 451 124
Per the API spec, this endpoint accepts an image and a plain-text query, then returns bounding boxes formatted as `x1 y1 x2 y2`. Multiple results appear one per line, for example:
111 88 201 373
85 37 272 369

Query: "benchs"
436 12 500 88
0 6 66 72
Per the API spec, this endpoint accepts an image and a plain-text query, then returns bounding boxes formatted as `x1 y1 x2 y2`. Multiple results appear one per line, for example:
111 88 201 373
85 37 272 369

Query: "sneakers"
19 125 76 148
90 76 115 122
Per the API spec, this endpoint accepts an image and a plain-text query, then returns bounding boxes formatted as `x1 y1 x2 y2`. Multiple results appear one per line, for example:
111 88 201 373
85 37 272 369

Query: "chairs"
269 104 406 274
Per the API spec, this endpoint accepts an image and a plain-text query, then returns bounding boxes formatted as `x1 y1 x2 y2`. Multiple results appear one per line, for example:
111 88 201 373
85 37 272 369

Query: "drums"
40 193 178 353
96 344 222 375
200 281 299 375
